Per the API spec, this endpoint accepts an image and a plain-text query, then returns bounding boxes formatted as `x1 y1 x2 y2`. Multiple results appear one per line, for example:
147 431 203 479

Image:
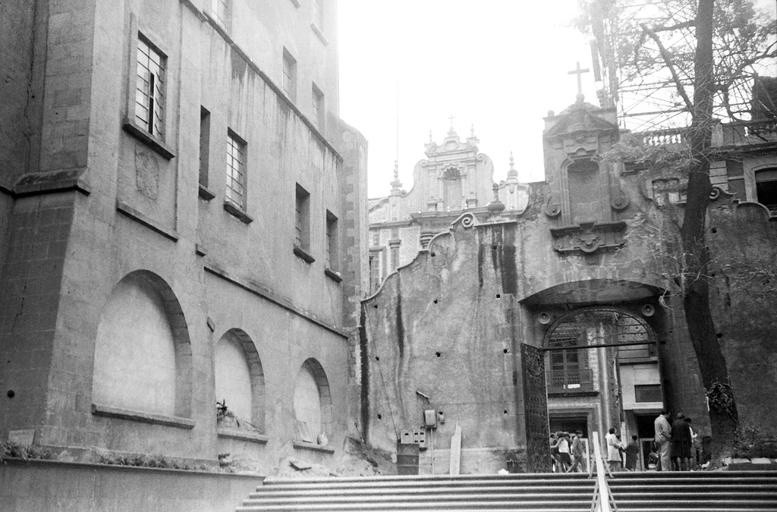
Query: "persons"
615 433 625 468
654 408 674 470
550 430 588 472
606 428 627 471
648 441 658 464
625 434 639 470
670 411 690 470
684 417 697 471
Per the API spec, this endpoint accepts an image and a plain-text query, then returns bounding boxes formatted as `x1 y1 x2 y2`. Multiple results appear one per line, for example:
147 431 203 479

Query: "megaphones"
641 304 655 317
537 311 551 325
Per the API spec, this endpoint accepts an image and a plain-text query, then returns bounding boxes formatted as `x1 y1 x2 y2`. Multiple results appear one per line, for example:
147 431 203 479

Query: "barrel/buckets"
397 441 418 476
